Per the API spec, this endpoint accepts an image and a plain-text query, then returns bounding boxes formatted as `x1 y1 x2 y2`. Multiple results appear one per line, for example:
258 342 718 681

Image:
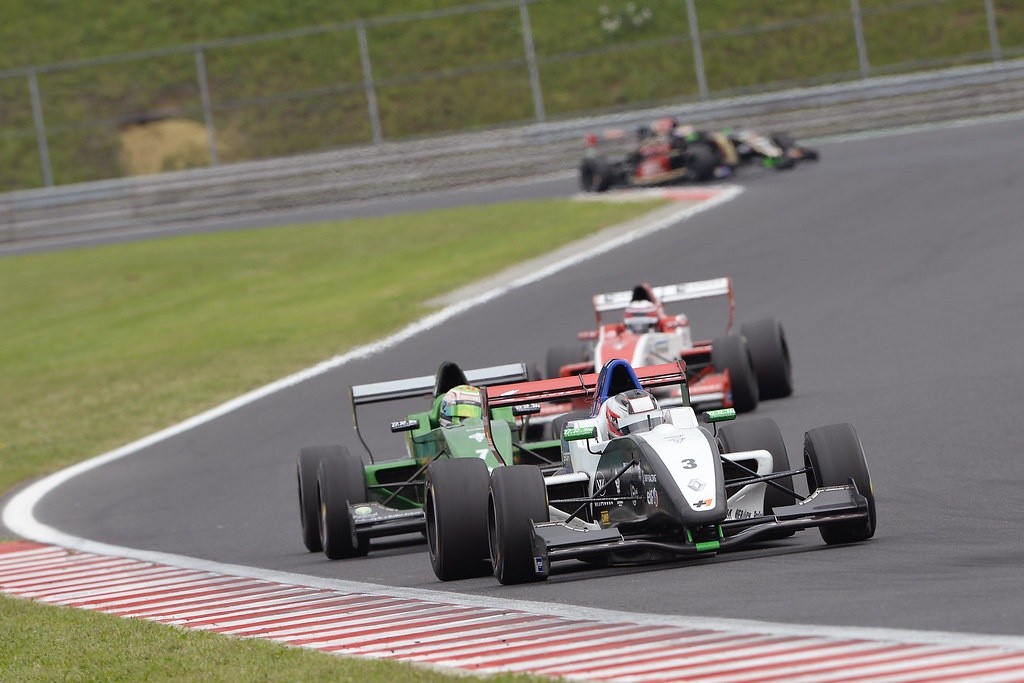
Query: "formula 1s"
423 358 880 587
477 271 792 442
293 362 566 561
575 115 820 196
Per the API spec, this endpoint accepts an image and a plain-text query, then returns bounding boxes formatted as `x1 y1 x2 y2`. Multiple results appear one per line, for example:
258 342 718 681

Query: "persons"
440 385 482 428
607 389 664 440
624 300 660 332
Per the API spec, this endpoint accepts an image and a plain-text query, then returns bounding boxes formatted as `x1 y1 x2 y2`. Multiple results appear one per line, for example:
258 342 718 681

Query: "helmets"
442 387 483 420
623 299 660 325
599 389 665 430
